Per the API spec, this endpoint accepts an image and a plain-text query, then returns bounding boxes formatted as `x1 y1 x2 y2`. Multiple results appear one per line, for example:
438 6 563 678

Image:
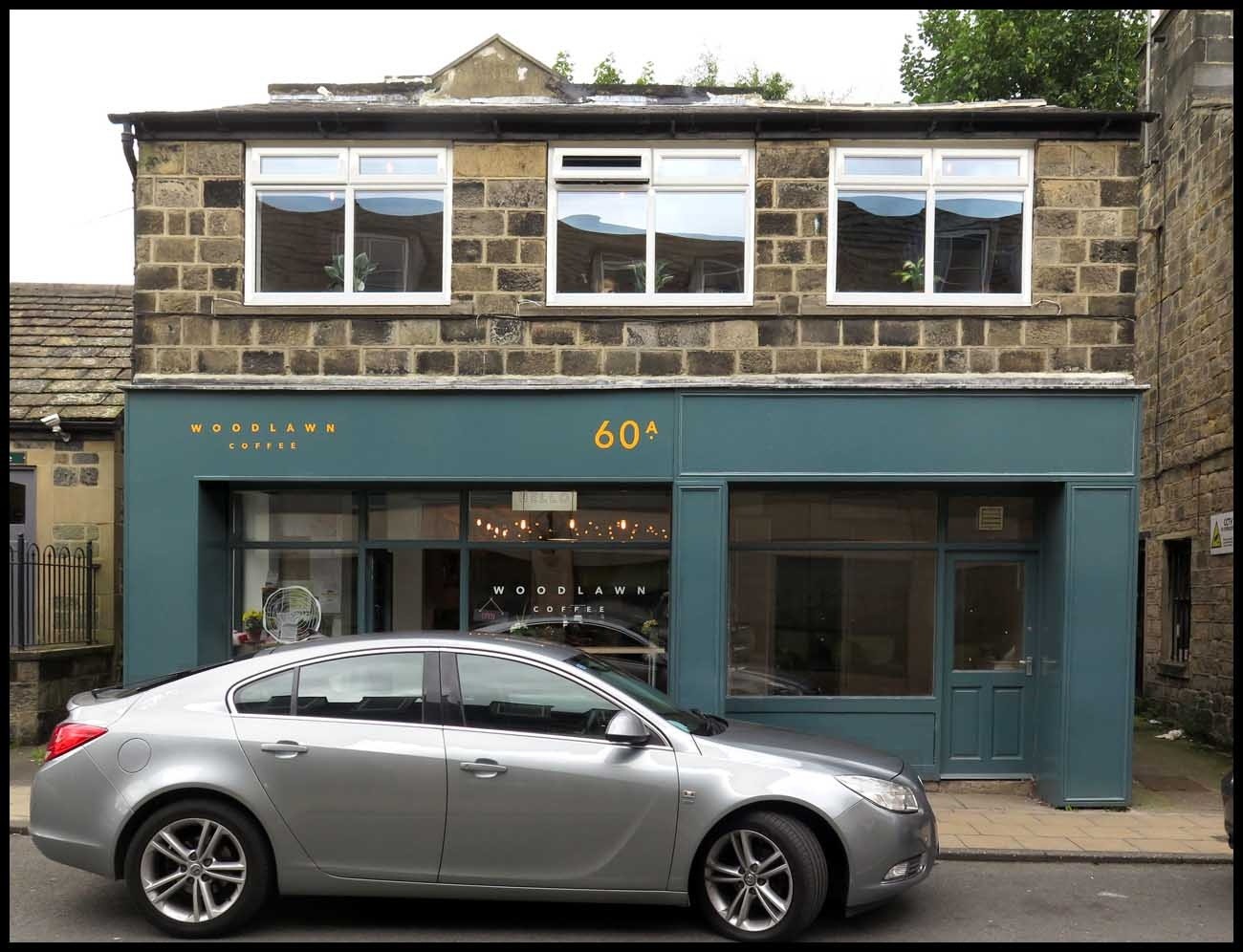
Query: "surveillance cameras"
41 413 62 435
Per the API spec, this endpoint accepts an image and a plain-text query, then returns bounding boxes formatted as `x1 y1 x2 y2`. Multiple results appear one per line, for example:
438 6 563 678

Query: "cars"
28 630 939 941
474 611 828 696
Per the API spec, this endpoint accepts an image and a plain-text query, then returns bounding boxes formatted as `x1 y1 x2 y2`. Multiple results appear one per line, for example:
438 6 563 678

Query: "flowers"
243 610 265 628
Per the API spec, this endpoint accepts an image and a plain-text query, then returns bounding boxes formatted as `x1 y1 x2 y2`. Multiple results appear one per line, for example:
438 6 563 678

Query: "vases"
245 627 261 643
